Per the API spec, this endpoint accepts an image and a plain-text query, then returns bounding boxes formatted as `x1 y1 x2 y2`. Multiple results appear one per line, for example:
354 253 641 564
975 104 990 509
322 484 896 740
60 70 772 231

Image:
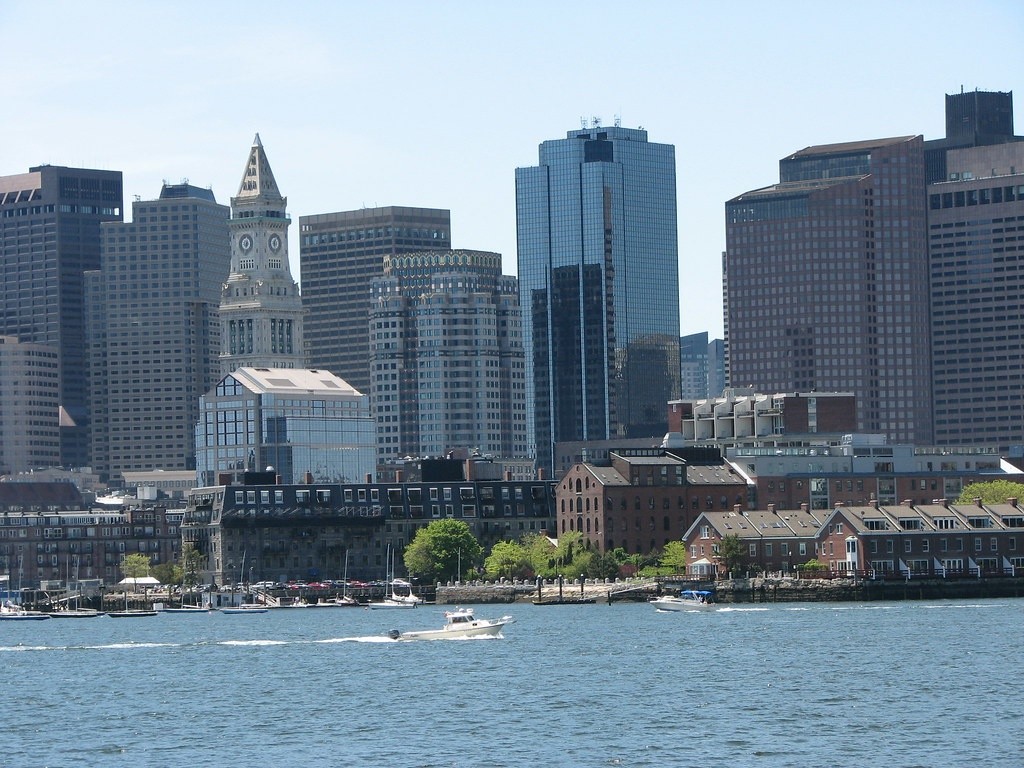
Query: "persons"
58 604 68 610
698 594 714 604
86 596 117 612
767 570 779 578
140 579 292 594
0 603 18 612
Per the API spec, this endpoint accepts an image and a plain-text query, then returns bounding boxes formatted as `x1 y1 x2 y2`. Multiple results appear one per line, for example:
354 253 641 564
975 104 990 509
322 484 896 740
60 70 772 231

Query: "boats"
648 595 718 613
0 600 104 620
151 608 209 613
679 590 717 607
316 597 342 607
387 607 514 642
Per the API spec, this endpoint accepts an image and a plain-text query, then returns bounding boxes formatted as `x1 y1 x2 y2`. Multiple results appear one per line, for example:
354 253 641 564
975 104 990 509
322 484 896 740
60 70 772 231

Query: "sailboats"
107 554 160 618
218 549 270 613
368 543 427 610
335 551 358 604
